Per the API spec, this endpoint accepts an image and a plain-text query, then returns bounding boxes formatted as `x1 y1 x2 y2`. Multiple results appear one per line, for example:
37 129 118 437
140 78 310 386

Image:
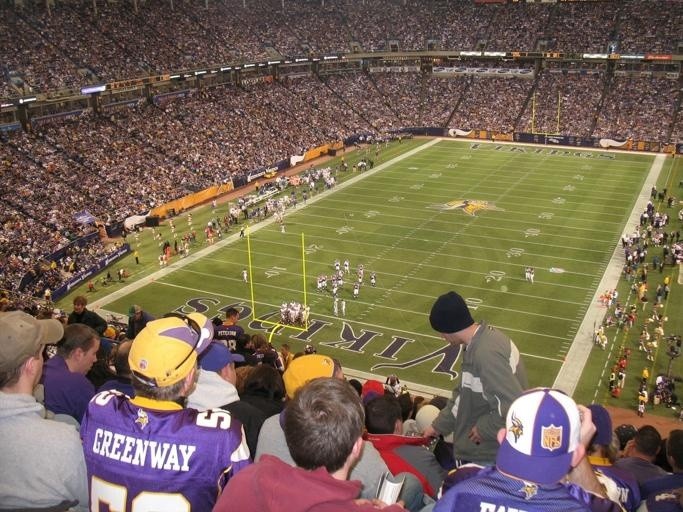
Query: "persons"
0 0 683 511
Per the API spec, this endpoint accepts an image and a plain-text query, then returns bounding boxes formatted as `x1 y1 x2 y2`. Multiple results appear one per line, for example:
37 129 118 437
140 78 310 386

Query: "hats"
0 311 64 373
429 291 474 333
126 305 142 318
362 380 384 406
102 327 116 338
127 312 214 388
212 318 222 327
415 405 441 432
495 387 638 486
197 339 245 373
282 354 335 400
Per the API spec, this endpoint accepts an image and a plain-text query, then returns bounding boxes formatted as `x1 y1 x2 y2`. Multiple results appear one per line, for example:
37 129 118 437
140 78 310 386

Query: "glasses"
162 313 201 370
